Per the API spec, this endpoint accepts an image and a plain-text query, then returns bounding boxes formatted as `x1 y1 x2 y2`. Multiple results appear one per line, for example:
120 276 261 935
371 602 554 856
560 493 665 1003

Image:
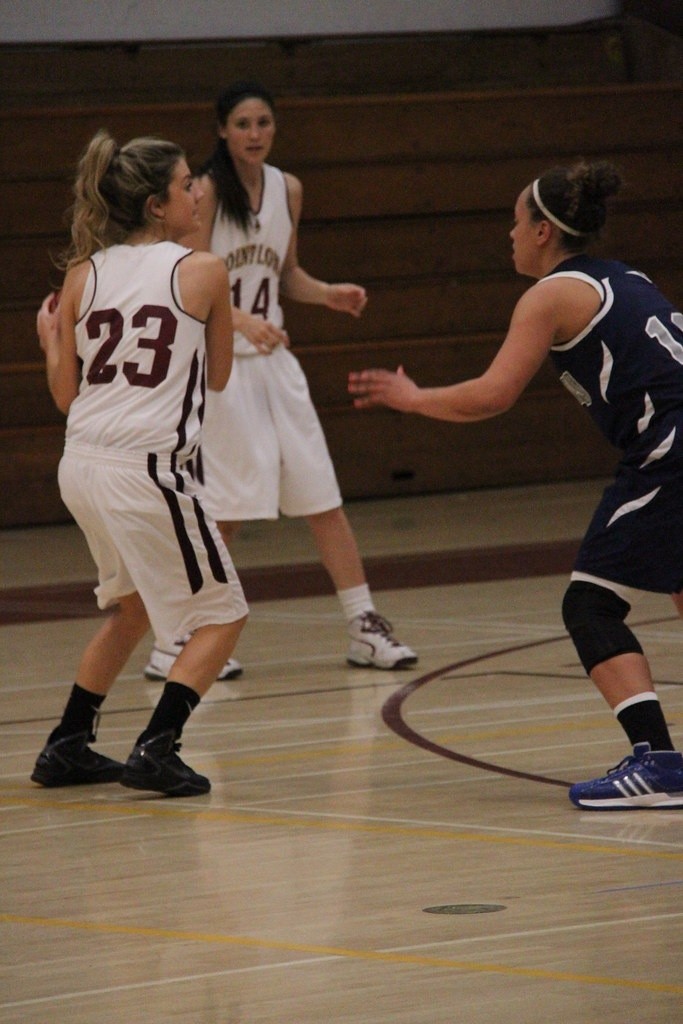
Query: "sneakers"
145 633 244 681
120 732 211 795
346 612 418 669
570 742 683 809
30 734 124 787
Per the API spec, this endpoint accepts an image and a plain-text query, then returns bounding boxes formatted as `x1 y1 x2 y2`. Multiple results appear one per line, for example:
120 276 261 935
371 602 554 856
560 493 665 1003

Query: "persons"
345 157 683 811
29 126 250 799
143 79 417 680
